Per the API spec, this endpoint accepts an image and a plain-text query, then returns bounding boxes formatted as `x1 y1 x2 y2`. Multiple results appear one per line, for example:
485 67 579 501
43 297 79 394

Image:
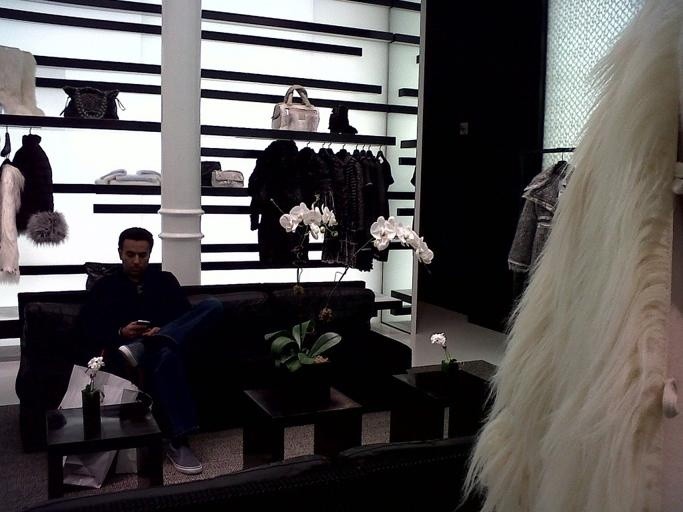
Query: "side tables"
42 405 163 497
244 386 363 463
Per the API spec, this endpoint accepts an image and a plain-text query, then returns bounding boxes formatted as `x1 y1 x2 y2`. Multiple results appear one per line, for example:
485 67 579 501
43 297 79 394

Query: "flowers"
262 202 436 364
429 332 452 360
83 354 103 389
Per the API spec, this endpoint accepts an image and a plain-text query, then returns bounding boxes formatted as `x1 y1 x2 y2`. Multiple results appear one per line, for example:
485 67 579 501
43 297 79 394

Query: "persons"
88 227 224 475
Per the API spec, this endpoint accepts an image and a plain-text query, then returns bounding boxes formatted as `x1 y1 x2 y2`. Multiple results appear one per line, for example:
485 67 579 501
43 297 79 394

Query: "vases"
273 366 331 411
81 388 101 439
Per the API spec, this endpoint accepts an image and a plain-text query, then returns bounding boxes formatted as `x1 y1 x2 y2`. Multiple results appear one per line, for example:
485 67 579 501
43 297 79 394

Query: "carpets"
0 358 497 512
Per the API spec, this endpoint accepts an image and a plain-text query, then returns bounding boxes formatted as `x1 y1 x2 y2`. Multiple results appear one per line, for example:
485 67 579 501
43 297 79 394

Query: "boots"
328 105 358 135
0 45 45 116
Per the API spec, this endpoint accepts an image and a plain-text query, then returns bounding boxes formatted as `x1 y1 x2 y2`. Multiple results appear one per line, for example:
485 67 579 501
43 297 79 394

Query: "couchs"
15 280 410 415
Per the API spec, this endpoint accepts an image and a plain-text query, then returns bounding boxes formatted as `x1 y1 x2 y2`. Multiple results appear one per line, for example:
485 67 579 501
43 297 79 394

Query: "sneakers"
118 341 146 369
166 444 204 475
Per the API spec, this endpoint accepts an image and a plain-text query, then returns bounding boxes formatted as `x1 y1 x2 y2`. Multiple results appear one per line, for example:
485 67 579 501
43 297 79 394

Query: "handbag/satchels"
62 86 121 121
57 364 141 492
271 101 320 132
211 169 244 188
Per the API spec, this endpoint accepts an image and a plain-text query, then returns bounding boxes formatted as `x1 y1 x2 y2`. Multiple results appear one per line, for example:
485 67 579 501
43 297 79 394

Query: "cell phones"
134 318 151 329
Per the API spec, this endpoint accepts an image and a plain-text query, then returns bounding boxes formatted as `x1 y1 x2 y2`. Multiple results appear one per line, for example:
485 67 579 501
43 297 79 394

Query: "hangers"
1 109 15 166
551 149 568 173
23 114 38 143
272 132 385 158
